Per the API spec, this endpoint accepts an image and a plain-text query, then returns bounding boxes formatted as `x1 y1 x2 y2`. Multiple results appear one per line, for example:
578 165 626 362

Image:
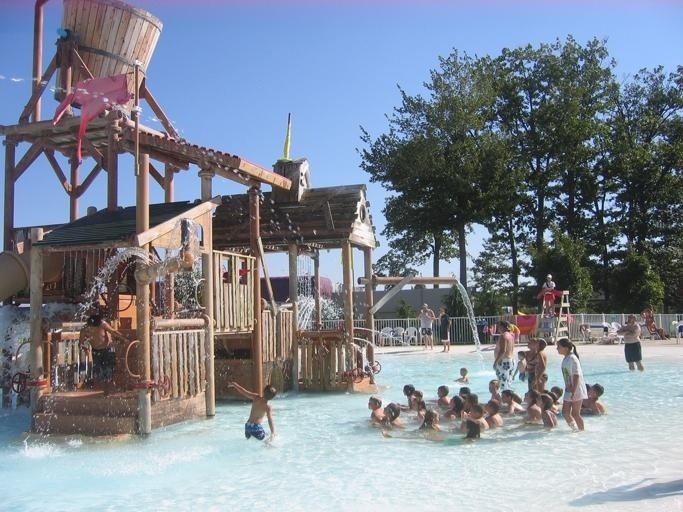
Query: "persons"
228 381 277 440
78 315 131 390
617 304 671 370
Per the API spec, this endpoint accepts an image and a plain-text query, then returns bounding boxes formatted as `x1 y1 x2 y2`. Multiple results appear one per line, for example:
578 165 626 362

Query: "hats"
546 273 553 279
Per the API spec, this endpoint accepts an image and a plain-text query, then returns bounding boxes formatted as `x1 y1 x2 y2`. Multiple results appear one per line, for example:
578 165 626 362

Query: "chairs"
578 318 683 346
391 326 405 347
378 327 390 345
417 327 433 346
405 327 417 345
